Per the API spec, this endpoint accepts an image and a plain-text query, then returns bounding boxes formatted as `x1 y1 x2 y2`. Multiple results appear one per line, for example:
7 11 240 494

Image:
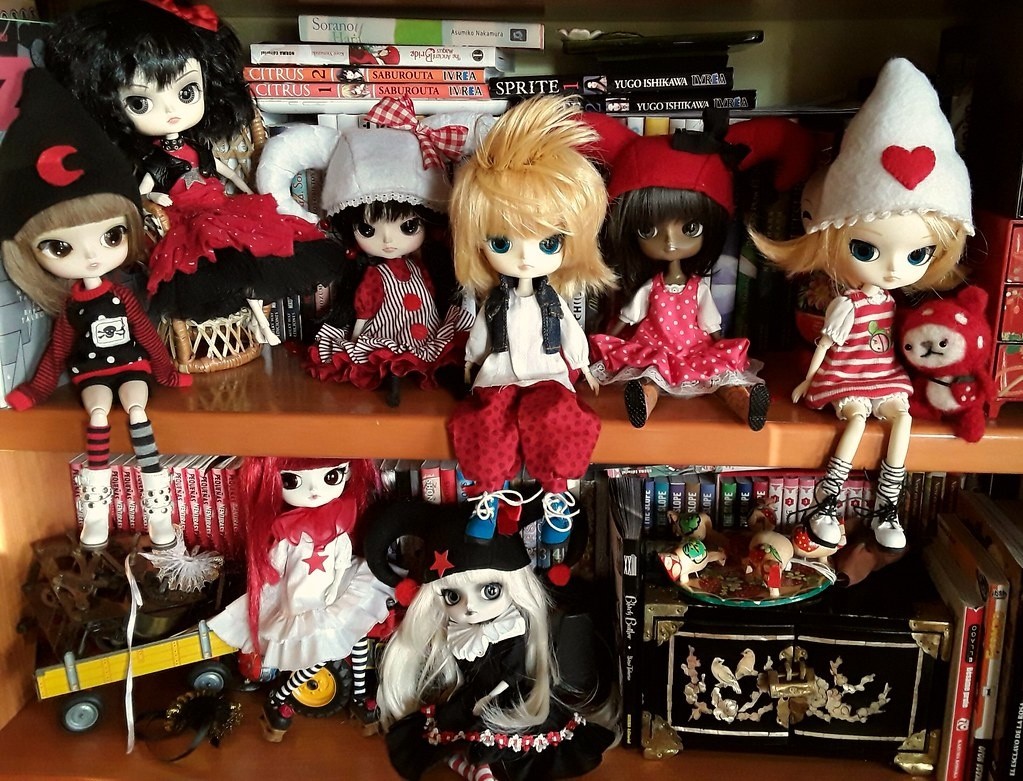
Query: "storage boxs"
958 214 1023 423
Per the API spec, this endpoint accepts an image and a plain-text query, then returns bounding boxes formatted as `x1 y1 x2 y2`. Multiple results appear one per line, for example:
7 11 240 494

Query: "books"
614 116 705 135
606 467 1022 781
67 452 593 569
242 15 543 99
489 66 756 114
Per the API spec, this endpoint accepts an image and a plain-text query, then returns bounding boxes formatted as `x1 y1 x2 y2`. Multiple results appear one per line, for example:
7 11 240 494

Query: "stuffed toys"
896 286 998 443
835 542 910 587
743 498 847 596
659 512 725 586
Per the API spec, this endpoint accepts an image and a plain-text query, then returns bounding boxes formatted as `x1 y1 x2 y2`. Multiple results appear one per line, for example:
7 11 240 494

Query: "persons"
374 530 616 781
69 0 329 347
0 66 192 553
448 93 613 549
746 56 975 552
205 457 396 741
256 111 495 405
557 109 809 430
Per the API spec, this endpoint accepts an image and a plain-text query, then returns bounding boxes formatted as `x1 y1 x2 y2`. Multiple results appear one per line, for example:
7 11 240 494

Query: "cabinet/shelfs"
0 363 1023 780
